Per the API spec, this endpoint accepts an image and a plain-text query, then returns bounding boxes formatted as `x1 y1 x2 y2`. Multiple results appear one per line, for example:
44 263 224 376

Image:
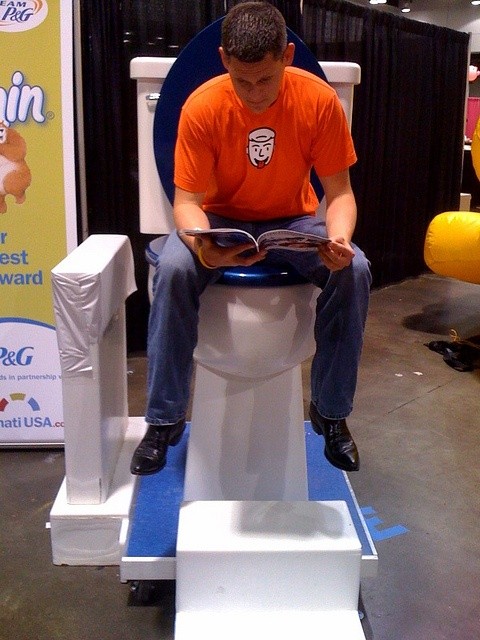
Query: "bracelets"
198 246 217 270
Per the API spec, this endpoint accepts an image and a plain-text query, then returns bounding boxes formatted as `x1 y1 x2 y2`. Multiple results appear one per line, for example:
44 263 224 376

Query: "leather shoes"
130 419 186 476
309 402 359 471
